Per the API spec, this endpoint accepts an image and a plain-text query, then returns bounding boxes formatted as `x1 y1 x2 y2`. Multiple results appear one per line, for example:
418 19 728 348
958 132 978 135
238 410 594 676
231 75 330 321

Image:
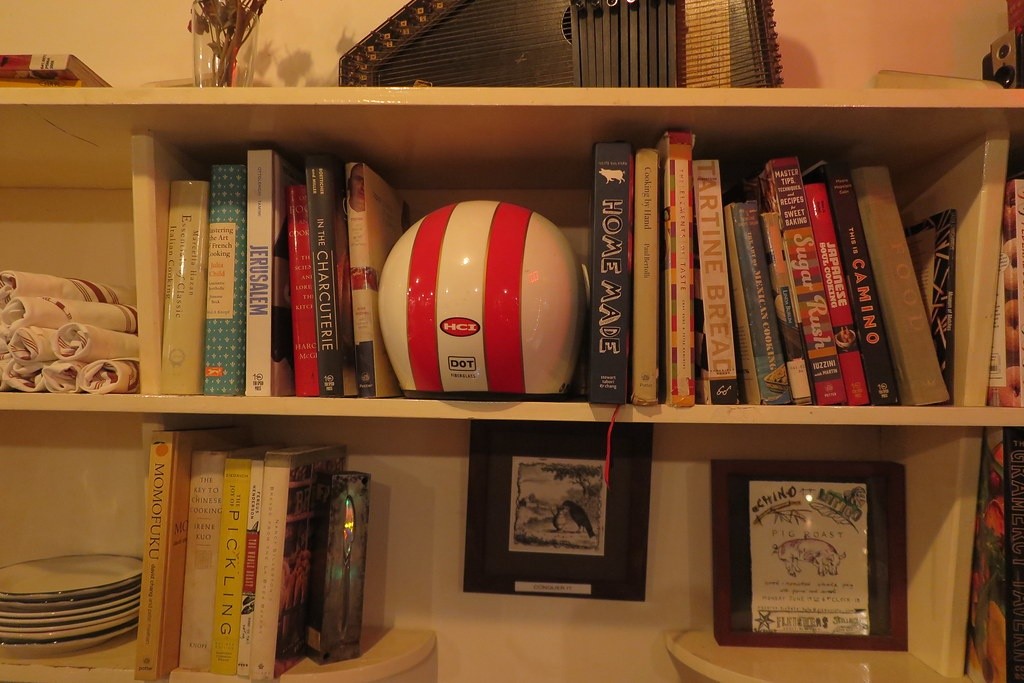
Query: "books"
585 126 1023 410
133 413 348 683
0 53 115 88
131 132 403 399
966 426 1024 683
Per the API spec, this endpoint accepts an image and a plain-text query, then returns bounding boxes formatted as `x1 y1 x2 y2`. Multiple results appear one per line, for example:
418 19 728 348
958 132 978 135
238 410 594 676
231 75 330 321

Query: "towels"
76 357 141 395
49 322 140 363
3 359 58 393
0 295 139 337
41 359 90 395
6 325 60 364
0 270 121 308
0 336 13 392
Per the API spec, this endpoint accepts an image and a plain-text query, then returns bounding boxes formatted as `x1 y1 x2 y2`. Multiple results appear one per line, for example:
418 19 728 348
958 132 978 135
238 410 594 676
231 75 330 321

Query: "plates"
0 554 143 660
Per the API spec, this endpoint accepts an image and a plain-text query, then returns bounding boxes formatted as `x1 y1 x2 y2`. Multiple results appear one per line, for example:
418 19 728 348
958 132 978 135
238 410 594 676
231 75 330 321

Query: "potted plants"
189 0 267 89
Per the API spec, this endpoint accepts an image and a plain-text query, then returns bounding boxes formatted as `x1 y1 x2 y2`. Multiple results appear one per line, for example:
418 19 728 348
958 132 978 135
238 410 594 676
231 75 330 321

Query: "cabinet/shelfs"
0 86 1024 682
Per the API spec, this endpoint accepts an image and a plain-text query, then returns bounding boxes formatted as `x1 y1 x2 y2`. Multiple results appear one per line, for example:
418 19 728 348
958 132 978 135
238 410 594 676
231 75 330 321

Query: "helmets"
378 199 585 399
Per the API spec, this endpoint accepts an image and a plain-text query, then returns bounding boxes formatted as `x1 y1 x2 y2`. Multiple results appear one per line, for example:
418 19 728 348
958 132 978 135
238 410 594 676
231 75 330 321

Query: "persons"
347 162 364 212
278 533 306 610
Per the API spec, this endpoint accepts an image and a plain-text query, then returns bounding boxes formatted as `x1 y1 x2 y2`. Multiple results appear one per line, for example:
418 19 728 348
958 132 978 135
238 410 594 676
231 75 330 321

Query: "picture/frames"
464 419 652 601
710 454 908 652
